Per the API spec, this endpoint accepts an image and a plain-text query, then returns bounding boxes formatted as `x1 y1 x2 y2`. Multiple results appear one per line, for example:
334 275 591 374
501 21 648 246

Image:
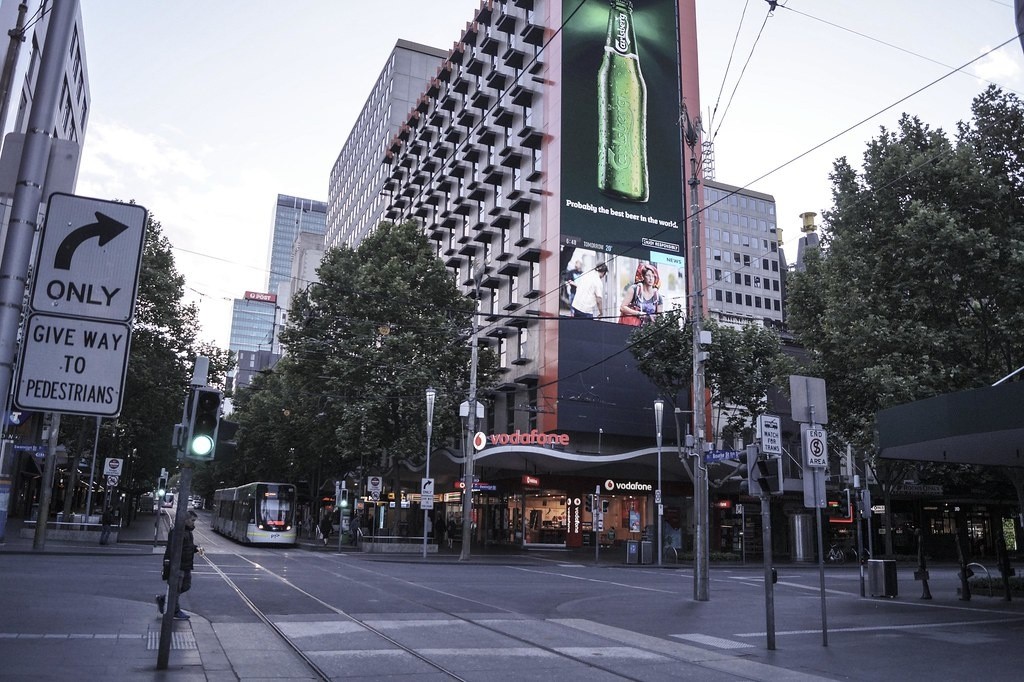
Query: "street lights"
652 397 666 565
422 386 438 559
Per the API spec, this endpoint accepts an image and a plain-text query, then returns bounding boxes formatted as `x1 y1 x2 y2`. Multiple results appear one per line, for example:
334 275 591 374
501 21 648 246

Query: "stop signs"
104 458 123 475
367 476 382 492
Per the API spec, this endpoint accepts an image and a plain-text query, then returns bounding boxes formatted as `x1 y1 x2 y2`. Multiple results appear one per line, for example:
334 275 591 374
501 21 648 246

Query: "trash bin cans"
640 541 652 565
379 529 389 543
867 559 898 598
626 540 639 564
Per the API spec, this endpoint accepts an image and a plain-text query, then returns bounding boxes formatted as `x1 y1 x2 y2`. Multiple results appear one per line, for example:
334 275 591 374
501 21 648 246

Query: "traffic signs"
27 190 148 325
421 477 434 495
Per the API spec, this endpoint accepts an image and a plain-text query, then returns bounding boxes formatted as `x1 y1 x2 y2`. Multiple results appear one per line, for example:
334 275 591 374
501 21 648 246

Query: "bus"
210 481 299 546
161 493 174 508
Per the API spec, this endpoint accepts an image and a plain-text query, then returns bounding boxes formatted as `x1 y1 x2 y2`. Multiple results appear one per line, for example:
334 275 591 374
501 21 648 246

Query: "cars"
188 495 204 510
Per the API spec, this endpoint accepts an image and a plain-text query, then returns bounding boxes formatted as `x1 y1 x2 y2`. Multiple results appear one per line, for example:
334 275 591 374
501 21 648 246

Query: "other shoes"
156 594 166 614
173 610 191 620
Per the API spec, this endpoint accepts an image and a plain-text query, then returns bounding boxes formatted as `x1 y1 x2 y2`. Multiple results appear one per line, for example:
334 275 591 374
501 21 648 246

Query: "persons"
368 516 378 542
156 511 199 620
99 506 113 545
55 493 82 512
572 263 609 320
320 515 334 546
435 516 446 545
349 515 360 547
567 261 583 316
618 265 664 327
426 518 433 544
632 523 637 530
446 525 453 548
305 515 314 540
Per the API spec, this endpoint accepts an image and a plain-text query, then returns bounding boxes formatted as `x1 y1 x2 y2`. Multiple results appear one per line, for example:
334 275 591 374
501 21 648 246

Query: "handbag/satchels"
163 529 183 581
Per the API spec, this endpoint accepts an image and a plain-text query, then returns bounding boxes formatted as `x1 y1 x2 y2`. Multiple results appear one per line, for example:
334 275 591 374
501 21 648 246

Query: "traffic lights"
738 444 762 497
602 500 610 513
834 488 851 519
205 416 240 466
158 476 167 498
340 489 348 508
757 455 784 496
585 494 593 513
184 387 222 461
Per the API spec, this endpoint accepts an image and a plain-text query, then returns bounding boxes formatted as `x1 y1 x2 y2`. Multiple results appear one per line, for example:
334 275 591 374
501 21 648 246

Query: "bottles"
197 543 204 557
596 0 650 202
